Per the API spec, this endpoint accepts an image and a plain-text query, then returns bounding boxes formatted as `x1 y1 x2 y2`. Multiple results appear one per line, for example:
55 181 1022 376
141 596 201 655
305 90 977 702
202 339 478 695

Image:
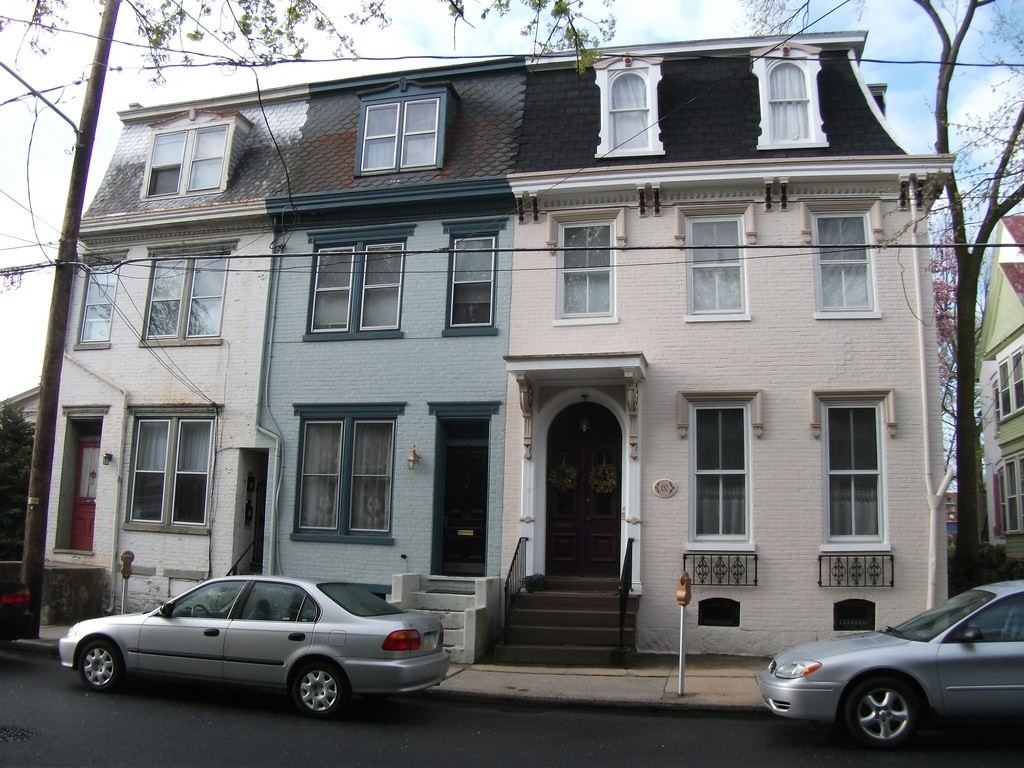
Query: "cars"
0 580 38 643
59 575 451 722
756 580 1024 752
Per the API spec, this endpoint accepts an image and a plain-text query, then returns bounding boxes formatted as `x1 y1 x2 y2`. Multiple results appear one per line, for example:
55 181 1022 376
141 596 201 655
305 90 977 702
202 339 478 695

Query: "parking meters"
120 550 135 613
676 571 691 696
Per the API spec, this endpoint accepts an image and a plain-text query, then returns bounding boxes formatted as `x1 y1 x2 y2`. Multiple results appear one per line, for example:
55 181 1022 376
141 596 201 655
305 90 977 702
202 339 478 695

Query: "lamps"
102 452 112 466
407 443 422 470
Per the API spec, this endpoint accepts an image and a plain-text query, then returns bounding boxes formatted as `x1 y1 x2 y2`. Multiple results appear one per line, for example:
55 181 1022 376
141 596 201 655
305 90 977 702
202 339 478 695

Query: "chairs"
249 599 275 621
